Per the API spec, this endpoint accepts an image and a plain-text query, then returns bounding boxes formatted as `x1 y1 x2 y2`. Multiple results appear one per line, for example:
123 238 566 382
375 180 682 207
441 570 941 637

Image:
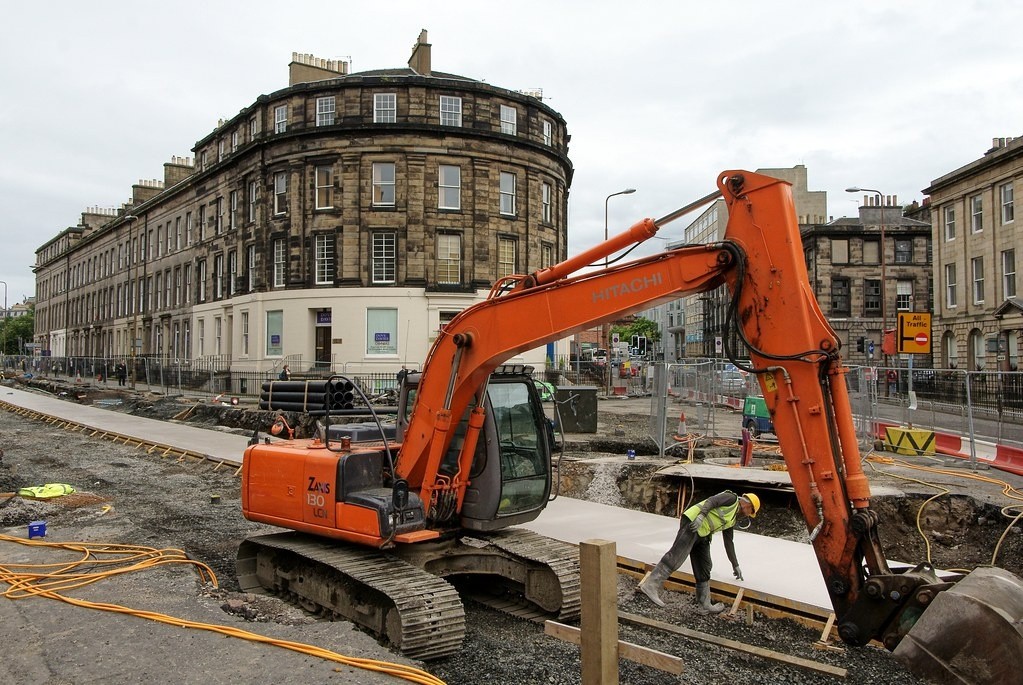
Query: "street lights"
604 188 636 270
0 281 7 355
845 186 889 399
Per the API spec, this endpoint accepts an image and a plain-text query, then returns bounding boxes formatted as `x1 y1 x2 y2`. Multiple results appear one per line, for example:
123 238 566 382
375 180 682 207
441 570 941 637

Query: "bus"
582 348 606 365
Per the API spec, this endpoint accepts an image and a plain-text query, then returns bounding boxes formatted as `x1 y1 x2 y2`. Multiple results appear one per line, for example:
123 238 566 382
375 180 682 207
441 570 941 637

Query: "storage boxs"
29 520 47 539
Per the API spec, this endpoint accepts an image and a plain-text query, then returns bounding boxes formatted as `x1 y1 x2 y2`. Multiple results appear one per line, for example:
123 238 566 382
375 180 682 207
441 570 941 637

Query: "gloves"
732 566 744 581
689 511 706 532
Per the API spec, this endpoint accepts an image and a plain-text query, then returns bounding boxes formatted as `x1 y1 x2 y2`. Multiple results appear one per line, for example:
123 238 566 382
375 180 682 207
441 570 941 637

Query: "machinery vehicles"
234 170 1023 685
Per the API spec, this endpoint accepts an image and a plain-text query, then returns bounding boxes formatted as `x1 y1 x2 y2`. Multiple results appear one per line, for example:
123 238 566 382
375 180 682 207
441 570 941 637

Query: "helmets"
743 493 760 518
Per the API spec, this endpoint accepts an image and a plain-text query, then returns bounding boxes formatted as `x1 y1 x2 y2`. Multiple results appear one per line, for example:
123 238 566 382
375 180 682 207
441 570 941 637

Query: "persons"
101 361 110 383
53 359 62 379
641 490 760 615
281 365 291 381
887 369 897 396
11 356 50 377
448 407 486 468
68 358 75 377
116 359 126 385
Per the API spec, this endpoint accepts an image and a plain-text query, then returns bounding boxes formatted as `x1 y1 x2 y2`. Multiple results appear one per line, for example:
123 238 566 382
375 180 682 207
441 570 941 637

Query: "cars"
610 358 762 399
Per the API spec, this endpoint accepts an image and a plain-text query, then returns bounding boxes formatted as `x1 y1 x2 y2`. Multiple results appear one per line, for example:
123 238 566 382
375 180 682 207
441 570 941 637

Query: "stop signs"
915 333 929 345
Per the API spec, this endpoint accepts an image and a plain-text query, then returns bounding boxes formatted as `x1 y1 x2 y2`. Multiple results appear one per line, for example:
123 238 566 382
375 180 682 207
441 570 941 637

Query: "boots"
640 561 673 607
695 580 724 615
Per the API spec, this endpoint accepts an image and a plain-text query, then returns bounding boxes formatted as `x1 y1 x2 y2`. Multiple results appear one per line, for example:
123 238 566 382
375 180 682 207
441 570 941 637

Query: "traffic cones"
673 413 691 441
666 380 674 397
75 370 82 384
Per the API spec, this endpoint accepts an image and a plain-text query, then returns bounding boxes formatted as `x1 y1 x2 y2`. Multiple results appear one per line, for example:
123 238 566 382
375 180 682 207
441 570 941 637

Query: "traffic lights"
857 337 865 354
632 336 638 348
637 338 646 355
